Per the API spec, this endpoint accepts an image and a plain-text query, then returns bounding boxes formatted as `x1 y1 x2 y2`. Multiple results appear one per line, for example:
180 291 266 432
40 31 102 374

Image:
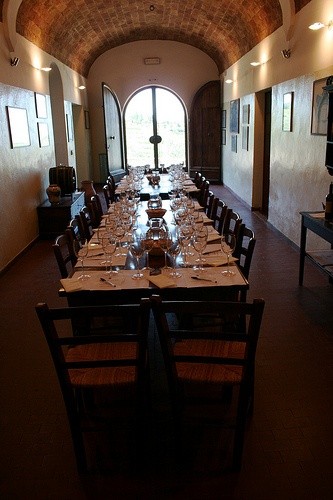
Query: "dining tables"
58 196 250 353
115 170 199 198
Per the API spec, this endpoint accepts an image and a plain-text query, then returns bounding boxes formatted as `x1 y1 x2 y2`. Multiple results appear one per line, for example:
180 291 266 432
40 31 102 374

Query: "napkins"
205 255 238 266
145 274 175 288
60 279 84 293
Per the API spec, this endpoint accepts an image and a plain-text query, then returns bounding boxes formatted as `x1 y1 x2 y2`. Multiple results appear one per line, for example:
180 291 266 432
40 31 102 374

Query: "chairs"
34 168 266 480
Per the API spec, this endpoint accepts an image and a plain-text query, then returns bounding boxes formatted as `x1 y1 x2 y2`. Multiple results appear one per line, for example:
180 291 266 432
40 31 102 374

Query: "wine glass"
97 164 209 279
72 238 90 281
220 233 237 277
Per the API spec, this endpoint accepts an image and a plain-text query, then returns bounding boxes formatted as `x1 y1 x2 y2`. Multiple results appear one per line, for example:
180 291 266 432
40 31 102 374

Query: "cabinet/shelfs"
37 191 85 245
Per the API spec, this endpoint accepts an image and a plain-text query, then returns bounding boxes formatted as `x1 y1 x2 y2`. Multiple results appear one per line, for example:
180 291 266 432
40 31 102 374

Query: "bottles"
151 171 160 185
148 191 162 209
325 183 333 220
146 218 166 239
80 180 96 205
46 184 61 202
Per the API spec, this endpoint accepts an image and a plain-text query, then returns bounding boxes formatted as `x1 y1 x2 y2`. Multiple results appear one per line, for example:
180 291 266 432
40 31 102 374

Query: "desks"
299 211 333 287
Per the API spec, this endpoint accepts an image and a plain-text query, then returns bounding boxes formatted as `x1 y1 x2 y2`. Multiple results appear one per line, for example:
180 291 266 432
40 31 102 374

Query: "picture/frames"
311 76 330 136
220 110 226 128
231 135 237 153
243 104 249 124
282 92 294 132
230 99 239 134
221 129 226 145
37 123 50 147
34 92 47 118
7 106 31 148
241 126 249 151
